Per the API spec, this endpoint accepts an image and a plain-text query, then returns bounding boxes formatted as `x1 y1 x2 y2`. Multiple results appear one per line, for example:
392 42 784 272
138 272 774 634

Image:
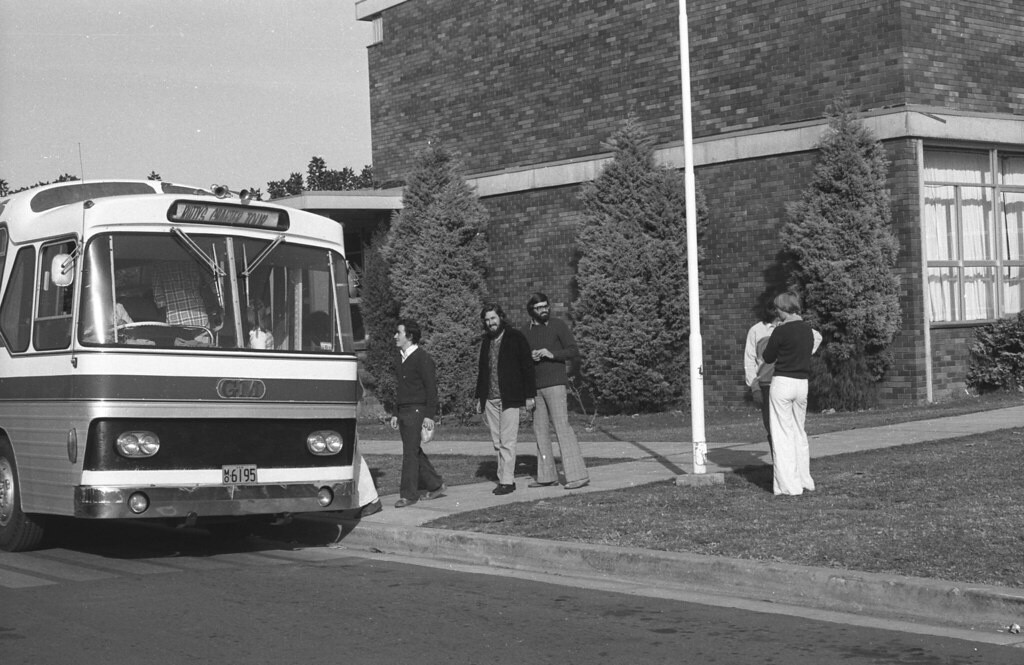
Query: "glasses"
533 304 551 311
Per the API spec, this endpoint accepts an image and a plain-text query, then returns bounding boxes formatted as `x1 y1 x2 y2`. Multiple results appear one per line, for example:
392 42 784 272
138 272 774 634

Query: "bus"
0 177 361 554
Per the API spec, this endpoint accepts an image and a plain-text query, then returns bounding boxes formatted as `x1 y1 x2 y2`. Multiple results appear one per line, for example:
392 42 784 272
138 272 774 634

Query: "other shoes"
563 477 590 488
394 497 417 507
358 499 382 517
493 482 516 495
527 480 559 488
420 482 447 501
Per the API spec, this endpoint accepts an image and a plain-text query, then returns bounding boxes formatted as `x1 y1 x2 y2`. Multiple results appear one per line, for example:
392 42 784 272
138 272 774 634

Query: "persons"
520 293 590 488
117 302 155 345
152 260 224 347
390 319 446 508
475 303 537 496
743 292 822 495
309 310 382 519
